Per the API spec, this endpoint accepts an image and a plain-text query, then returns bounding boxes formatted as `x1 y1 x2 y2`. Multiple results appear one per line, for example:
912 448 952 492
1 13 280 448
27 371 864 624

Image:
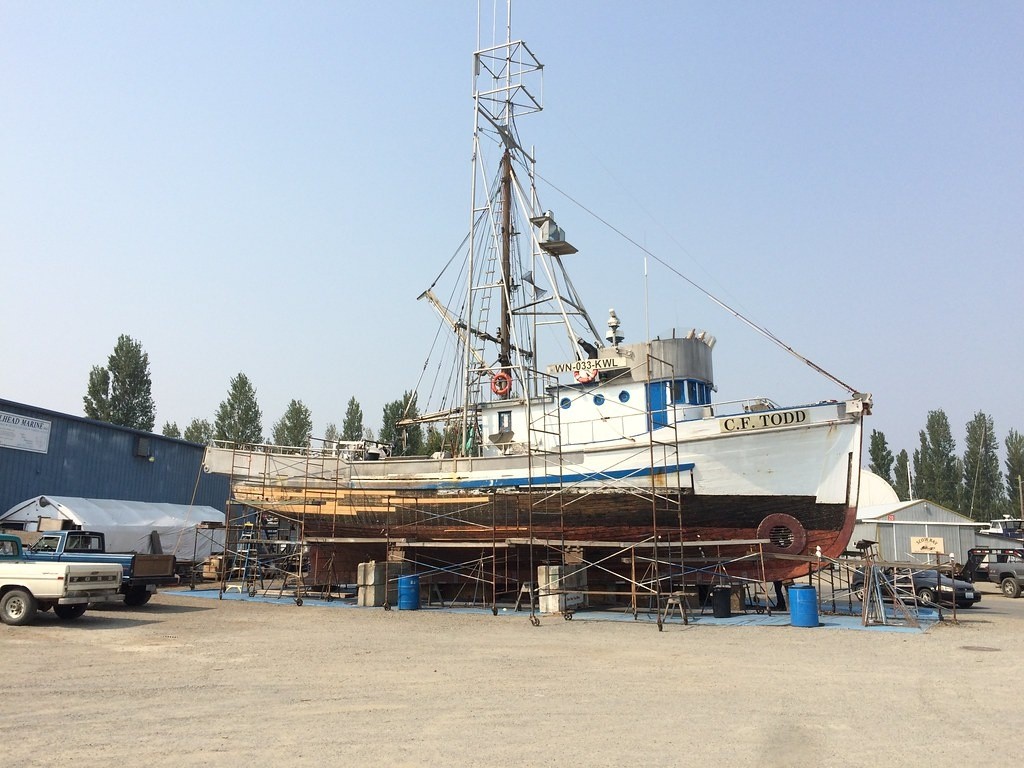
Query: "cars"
851 562 983 607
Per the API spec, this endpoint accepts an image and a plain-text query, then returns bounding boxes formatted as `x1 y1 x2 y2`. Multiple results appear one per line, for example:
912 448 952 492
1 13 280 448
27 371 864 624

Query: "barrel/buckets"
788 584 819 627
398 574 419 610
712 585 732 618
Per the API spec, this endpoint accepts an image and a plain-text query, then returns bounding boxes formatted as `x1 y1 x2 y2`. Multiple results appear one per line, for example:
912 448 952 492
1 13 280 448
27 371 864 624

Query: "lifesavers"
573 370 599 383
491 373 514 393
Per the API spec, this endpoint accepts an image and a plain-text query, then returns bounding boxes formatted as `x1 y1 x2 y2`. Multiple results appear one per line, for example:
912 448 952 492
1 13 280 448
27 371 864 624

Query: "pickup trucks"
979 514 1023 538
0 533 126 627
23 530 182 608
937 548 1024 599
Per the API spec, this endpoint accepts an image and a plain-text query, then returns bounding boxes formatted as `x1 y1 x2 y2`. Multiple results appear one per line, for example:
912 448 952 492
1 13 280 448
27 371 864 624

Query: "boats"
175 1 875 603
